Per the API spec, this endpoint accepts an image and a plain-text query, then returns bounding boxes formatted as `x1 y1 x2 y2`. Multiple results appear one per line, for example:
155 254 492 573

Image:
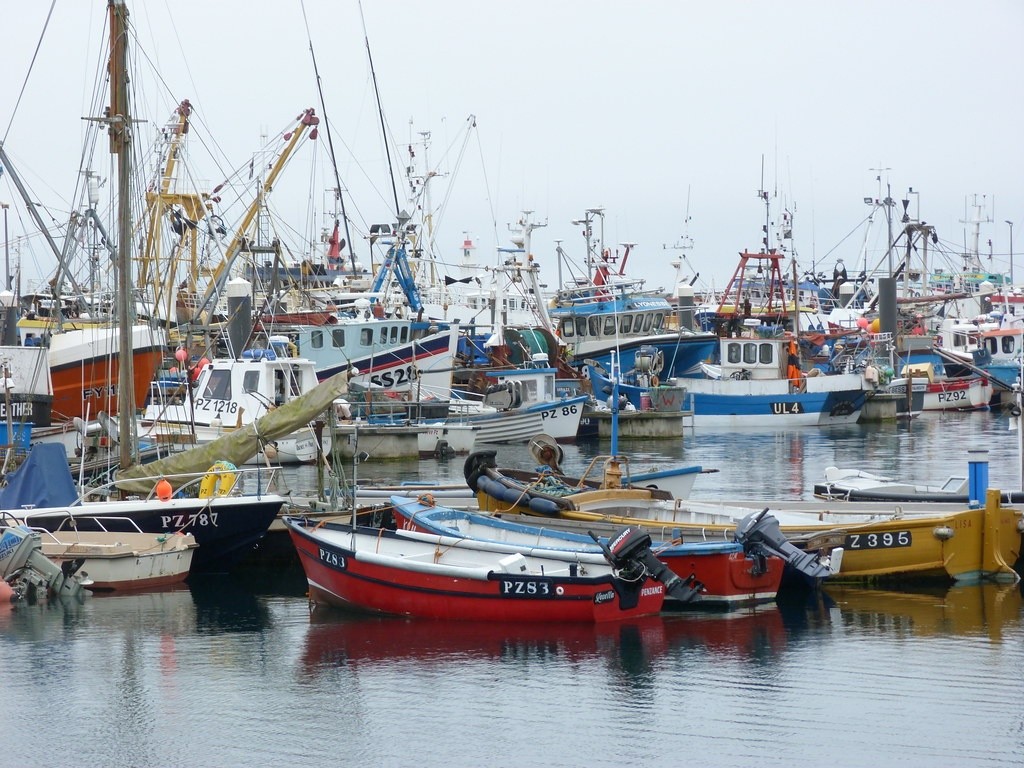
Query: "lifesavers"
198 460 238 497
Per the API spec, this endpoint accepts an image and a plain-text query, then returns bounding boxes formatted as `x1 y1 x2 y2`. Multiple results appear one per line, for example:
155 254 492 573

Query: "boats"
282 515 707 624
0 510 199 602
390 495 845 608
462 434 1024 582
0 2 1024 570
